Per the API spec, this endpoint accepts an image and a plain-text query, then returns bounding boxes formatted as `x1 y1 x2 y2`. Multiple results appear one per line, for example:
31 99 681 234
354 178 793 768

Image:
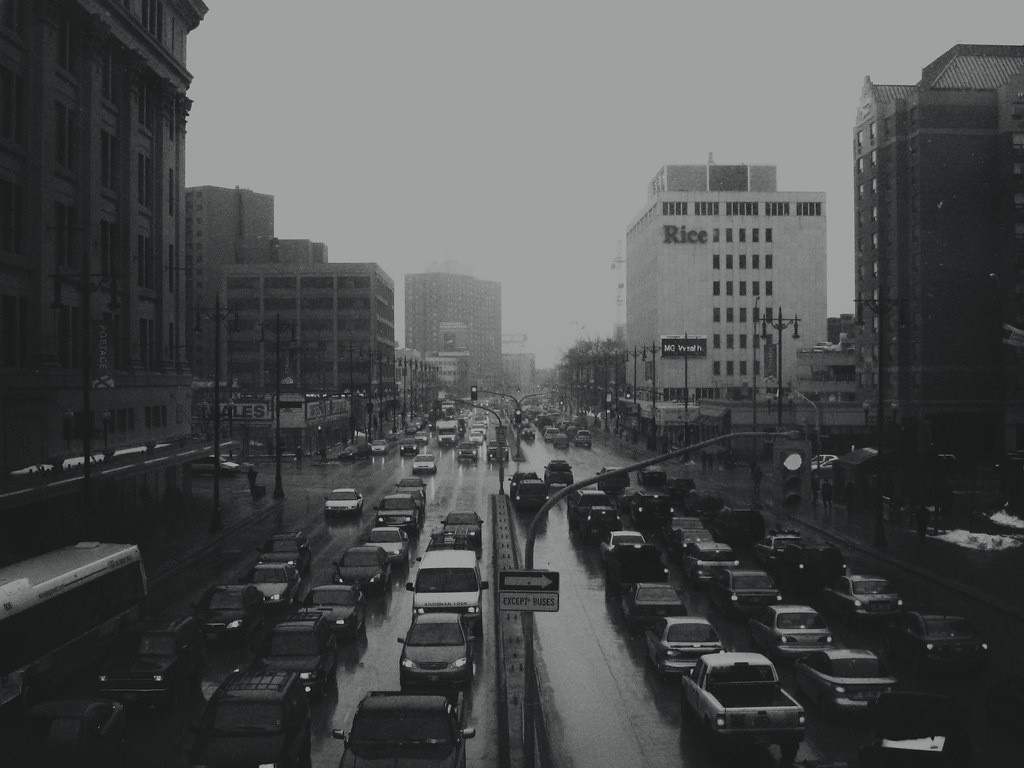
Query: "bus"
1 540 151 709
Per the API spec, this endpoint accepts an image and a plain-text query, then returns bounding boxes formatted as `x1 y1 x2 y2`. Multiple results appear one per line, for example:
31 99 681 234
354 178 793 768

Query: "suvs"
184 667 314 768
96 613 210 710
256 612 339 698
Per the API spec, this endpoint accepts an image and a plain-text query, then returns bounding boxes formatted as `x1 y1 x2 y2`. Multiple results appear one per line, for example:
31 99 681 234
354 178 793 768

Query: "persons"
248 467 257 492
701 451 714 470
811 473 820 505
821 478 833 509
915 505 930 536
296 445 302 465
319 447 325 462
752 465 763 490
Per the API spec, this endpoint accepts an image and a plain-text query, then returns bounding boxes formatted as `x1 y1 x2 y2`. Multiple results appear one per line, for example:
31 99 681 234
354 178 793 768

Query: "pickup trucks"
681 648 807 761
333 689 477 768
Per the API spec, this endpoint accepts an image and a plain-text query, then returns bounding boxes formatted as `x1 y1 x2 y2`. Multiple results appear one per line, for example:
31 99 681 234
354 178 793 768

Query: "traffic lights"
471 385 477 402
514 409 522 423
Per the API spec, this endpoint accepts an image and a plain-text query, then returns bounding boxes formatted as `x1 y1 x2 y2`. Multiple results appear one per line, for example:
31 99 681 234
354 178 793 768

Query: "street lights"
51 264 117 539
521 429 804 767
601 332 708 463
256 316 444 501
760 306 803 431
192 290 242 535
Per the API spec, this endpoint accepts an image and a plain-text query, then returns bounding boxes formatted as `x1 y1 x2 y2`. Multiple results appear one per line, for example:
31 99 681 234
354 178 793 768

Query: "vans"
405 550 491 632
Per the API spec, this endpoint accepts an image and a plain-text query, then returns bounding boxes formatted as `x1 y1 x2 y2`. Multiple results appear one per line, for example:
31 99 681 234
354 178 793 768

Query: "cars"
621 581 688 635
747 603 833 666
818 571 904 631
646 614 727 685
396 611 477 687
243 392 595 574
239 561 302 612
505 456 849 591
293 585 368 648
883 606 991 680
792 647 901 723
187 584 268 650
705 568 784 623
